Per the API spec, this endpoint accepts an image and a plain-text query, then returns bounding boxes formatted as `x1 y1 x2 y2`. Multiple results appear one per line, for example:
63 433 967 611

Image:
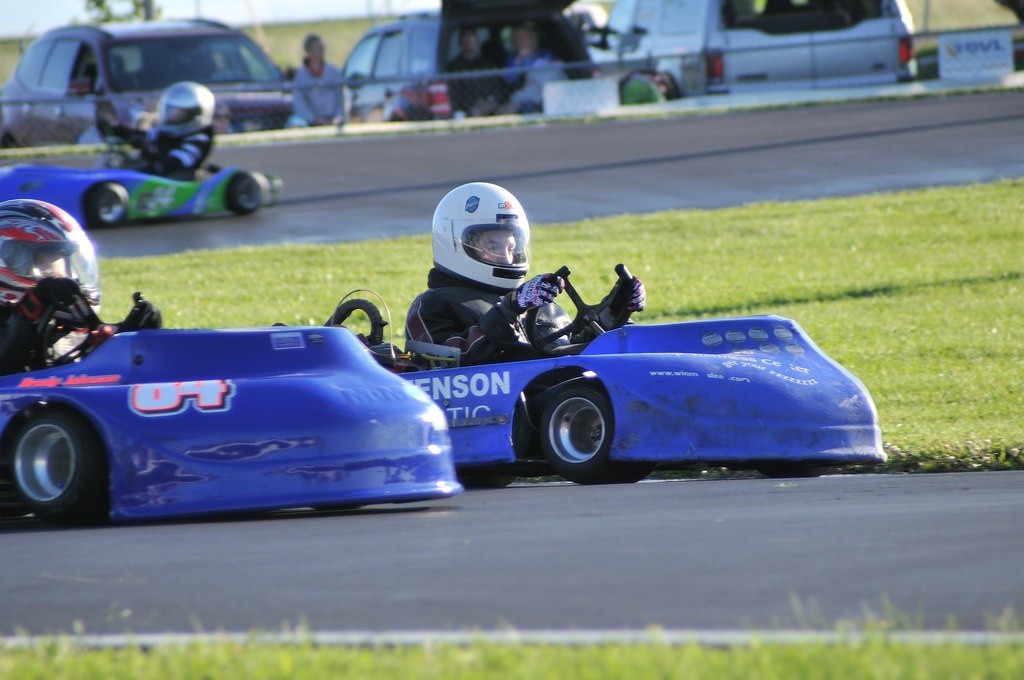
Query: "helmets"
0 198 101 309
156 80 217 134
431 182 531 289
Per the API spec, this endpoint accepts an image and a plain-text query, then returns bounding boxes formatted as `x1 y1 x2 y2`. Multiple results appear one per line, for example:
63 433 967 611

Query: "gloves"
504 273 565 315
123 291 162 332
605 276 643 321
19 277 82 322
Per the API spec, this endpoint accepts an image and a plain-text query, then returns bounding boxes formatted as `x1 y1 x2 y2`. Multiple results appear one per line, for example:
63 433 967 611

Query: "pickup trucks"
606 1 919 102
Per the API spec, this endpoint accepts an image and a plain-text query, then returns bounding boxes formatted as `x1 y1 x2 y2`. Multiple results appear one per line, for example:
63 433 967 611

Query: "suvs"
1 17 300 147
343 1 599 123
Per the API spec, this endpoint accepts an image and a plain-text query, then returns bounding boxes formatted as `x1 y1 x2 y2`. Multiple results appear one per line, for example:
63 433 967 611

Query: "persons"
498 21 555 115
448 26 511 115
0 198 163 374
401 181 644 366
292 34 345 126
97 81 215 183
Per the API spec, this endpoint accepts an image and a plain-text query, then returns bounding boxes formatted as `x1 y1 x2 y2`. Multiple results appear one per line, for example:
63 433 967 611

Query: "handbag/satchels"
620 67 676 104
